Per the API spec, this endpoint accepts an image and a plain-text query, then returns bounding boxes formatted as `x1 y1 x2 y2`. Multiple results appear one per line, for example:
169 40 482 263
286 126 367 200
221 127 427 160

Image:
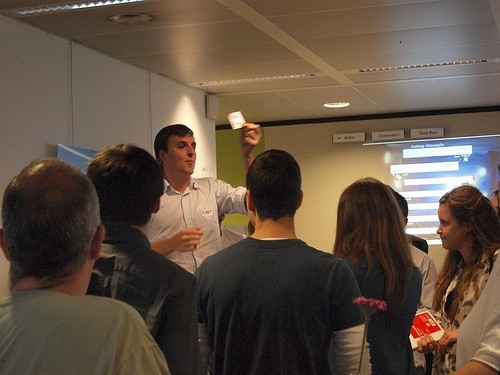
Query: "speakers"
206 95 220 119
